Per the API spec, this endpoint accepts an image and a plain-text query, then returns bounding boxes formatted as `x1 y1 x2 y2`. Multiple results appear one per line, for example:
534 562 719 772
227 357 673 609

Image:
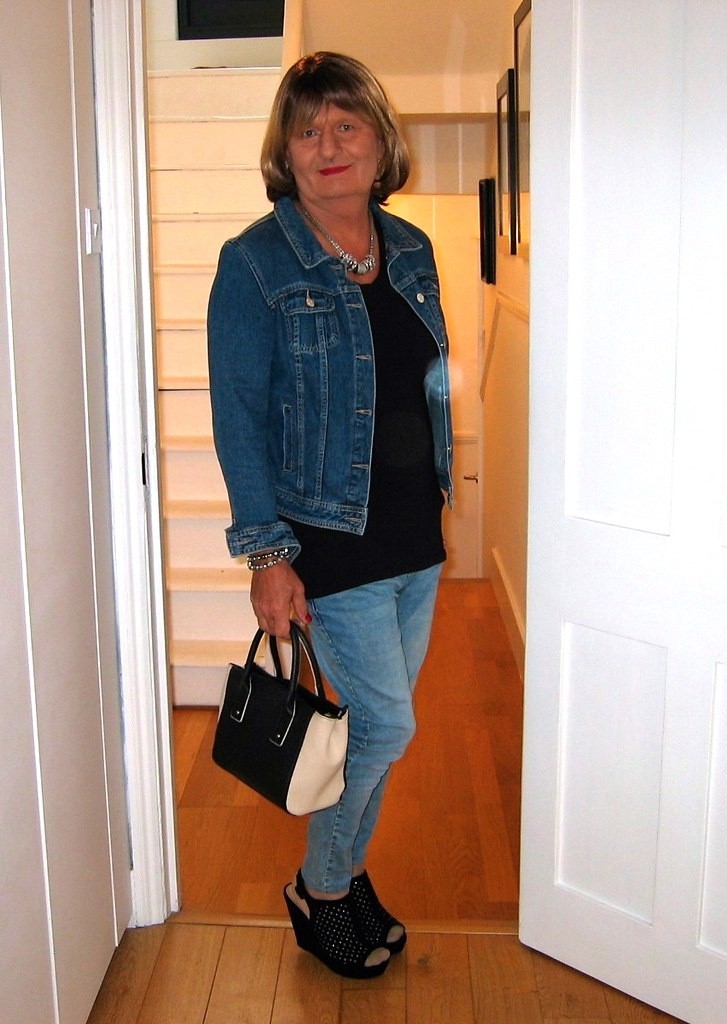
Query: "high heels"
283 867 390 979
349 868 407 955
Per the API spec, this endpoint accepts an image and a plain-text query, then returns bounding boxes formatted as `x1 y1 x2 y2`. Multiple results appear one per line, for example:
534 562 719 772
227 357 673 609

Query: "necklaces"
300 201 375 275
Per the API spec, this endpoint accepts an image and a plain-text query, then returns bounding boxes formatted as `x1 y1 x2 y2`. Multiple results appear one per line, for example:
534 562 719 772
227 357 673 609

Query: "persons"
205 51 454 976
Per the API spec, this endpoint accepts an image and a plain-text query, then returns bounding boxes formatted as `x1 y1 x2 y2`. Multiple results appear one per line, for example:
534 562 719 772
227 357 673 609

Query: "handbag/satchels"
211 622 349 815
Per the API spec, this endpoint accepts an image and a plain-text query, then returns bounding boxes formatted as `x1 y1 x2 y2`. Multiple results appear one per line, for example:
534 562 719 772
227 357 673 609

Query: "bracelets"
246 547 288 571
